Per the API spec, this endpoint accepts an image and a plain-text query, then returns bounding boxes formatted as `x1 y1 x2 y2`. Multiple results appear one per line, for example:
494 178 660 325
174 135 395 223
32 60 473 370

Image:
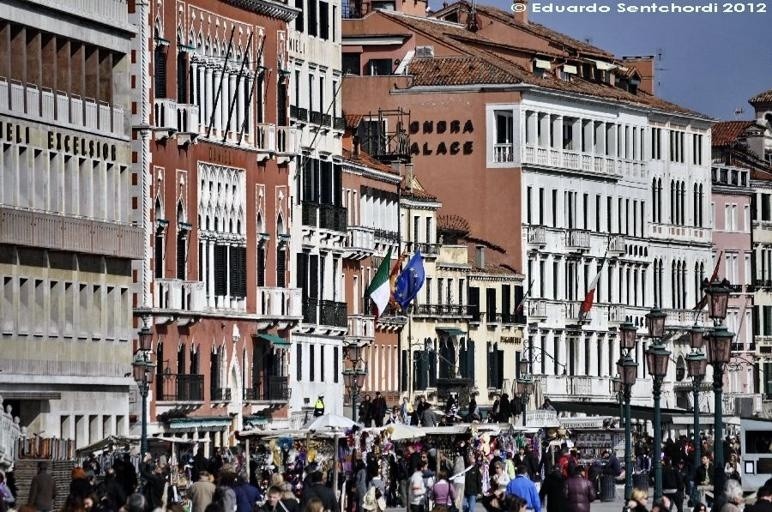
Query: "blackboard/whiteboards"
632 474 648 501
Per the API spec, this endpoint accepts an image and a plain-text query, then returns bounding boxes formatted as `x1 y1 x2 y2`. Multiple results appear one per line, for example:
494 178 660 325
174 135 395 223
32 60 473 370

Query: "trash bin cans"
600 474 615 503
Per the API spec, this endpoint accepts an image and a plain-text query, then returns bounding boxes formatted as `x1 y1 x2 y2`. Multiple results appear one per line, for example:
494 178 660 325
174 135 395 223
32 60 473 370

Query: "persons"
0 391 772 512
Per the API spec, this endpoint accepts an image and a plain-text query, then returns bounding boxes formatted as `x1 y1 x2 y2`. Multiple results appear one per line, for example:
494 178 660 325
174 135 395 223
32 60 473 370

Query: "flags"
366 251 391 318
394 249 425 312
578 260 603 322
388 258 401 311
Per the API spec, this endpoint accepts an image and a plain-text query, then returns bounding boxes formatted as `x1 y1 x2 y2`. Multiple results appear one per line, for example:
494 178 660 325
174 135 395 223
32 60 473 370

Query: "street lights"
616 312 640 512
643 304 672 506
683 320 708 487
514 355 536 426
132 324 156 459
703 276 737 504
613 374 625 428
341 340 367 425
